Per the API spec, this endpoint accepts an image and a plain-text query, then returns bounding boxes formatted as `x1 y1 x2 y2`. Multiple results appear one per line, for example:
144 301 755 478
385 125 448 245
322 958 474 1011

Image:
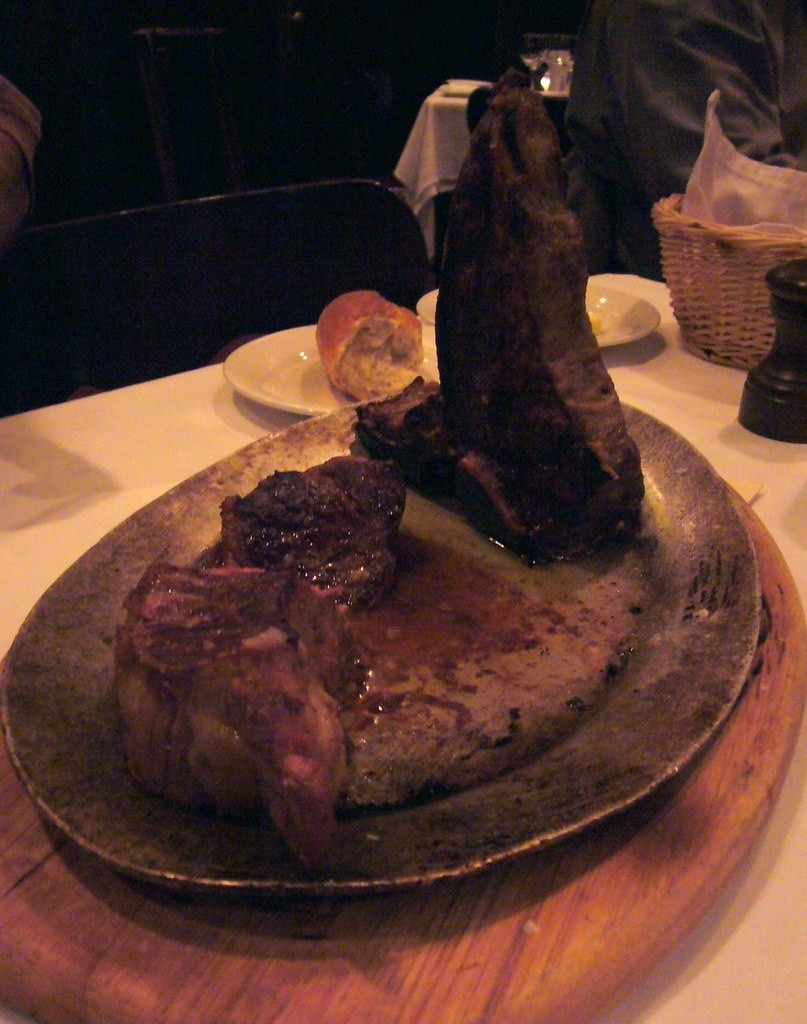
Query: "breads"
315 290 426 399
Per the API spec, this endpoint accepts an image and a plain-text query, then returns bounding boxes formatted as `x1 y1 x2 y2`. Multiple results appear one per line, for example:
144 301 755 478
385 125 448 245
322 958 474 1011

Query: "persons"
559 0 807 284
0 72 41 258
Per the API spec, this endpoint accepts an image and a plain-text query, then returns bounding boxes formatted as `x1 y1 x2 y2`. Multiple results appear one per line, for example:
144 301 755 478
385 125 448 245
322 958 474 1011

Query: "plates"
223 323 440 414
415 282 662 347
3 380 763 893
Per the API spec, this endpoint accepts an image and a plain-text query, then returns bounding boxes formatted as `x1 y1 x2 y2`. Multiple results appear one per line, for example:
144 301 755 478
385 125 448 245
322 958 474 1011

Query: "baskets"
650 193 807 371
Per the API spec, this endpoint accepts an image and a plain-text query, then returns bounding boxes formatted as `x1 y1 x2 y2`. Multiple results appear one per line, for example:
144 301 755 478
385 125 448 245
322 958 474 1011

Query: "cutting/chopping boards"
0 395 807 1024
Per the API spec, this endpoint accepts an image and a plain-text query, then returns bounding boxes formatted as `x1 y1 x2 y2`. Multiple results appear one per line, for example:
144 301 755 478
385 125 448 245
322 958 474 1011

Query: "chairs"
0 175 426 413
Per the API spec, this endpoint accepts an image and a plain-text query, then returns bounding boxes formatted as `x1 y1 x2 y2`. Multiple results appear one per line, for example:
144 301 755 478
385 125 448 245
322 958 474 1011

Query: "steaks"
218 455 407 611
107 560 346 876
354 85 644 566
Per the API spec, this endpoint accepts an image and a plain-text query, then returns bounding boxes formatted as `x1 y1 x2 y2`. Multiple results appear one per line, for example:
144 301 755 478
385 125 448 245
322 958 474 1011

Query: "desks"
1 273 806 1024
393 76 494 283
